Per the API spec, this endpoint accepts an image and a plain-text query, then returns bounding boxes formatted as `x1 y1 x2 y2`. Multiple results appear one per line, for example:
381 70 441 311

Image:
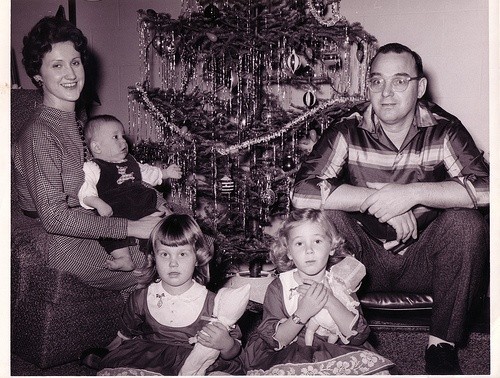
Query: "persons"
85 212 248 376
10 16 212 303
288 43 489 375
244 208 397 375
78 114 184 272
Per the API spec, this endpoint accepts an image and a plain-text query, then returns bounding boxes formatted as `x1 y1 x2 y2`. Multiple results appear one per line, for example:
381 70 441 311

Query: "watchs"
292 314 305 326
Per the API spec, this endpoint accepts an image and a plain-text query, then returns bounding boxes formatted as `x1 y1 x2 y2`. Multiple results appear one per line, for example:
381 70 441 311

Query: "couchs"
11 89 129 371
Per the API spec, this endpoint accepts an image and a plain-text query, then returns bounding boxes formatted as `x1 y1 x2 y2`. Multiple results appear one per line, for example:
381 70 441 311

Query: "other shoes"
424 343 464 375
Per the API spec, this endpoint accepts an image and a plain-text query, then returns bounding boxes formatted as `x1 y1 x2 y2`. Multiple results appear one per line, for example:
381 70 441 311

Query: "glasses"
366 77 419 92
